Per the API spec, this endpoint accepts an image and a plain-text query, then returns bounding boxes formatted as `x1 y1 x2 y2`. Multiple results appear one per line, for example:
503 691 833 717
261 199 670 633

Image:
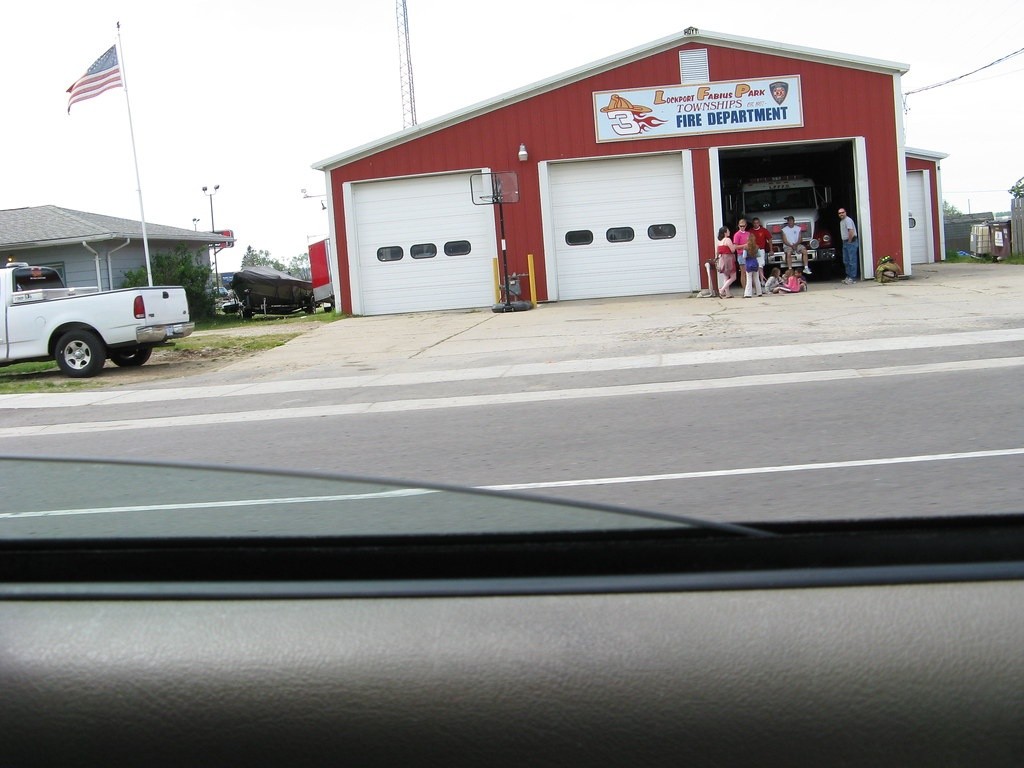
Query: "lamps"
518 143 528 162
321 200 327 210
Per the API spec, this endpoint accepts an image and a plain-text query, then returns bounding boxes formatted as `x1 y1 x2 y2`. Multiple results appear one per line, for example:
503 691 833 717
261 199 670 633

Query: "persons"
714 215 812 299
838 208 858 284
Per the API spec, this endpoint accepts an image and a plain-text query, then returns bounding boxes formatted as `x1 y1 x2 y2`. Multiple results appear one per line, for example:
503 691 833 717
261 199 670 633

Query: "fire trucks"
724 174 840 271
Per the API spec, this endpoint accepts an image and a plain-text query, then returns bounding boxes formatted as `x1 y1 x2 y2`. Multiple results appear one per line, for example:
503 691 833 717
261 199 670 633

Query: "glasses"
838 212 845 214
739 225 745 227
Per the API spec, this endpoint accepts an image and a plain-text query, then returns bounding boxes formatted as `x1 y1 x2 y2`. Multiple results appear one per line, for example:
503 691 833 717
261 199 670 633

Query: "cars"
212 287 229 298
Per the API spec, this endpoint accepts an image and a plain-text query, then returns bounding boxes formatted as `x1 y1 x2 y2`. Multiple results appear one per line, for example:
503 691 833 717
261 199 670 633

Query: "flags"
66 44 124 115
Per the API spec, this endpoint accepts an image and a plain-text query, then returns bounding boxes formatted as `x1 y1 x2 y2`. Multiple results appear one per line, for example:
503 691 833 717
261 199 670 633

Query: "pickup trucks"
0 260 195 379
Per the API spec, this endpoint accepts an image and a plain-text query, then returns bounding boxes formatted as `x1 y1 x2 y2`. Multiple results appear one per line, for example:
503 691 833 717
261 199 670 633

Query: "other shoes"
842 278 849 283
744 296 751 298
845 279 856 284
726 295 734 298
803 269 812 274
717 289 723 299
758 295 762 297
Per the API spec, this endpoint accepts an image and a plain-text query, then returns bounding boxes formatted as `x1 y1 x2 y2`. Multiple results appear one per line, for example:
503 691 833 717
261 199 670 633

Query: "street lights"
202 184 221 298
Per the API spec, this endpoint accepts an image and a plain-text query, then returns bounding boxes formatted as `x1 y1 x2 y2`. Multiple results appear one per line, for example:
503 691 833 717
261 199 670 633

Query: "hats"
784 216 793 220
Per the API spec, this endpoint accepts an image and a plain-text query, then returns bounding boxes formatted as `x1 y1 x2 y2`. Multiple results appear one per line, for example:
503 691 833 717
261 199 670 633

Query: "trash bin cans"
969 224 991 260
985 220 1012 262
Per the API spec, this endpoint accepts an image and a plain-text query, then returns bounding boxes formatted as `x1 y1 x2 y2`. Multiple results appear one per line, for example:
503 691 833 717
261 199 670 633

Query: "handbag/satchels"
717 257 723 273
